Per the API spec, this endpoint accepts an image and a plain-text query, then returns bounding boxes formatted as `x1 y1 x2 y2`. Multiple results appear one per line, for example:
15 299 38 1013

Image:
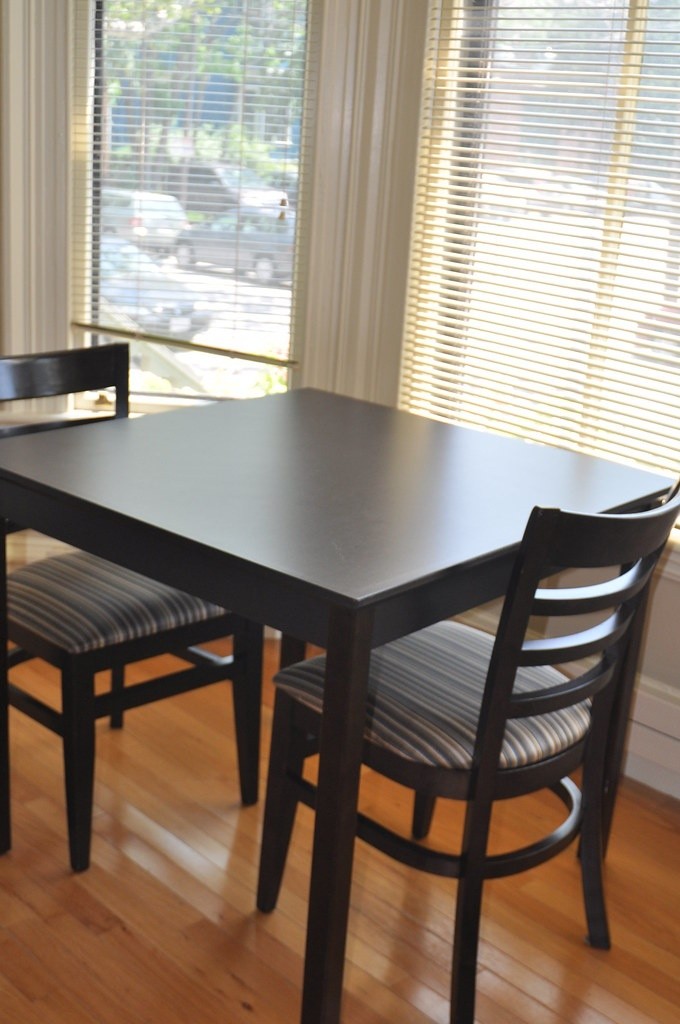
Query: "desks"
0 385 675 1024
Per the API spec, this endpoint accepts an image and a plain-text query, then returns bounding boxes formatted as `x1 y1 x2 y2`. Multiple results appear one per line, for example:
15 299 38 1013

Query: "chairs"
257 480 680 1024
0 339 265 871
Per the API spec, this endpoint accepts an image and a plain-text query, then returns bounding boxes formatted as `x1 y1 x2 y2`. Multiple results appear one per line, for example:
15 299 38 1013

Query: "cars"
97 234 211 351
96 188 186 256
167 162 289 215
173 206 299 287
